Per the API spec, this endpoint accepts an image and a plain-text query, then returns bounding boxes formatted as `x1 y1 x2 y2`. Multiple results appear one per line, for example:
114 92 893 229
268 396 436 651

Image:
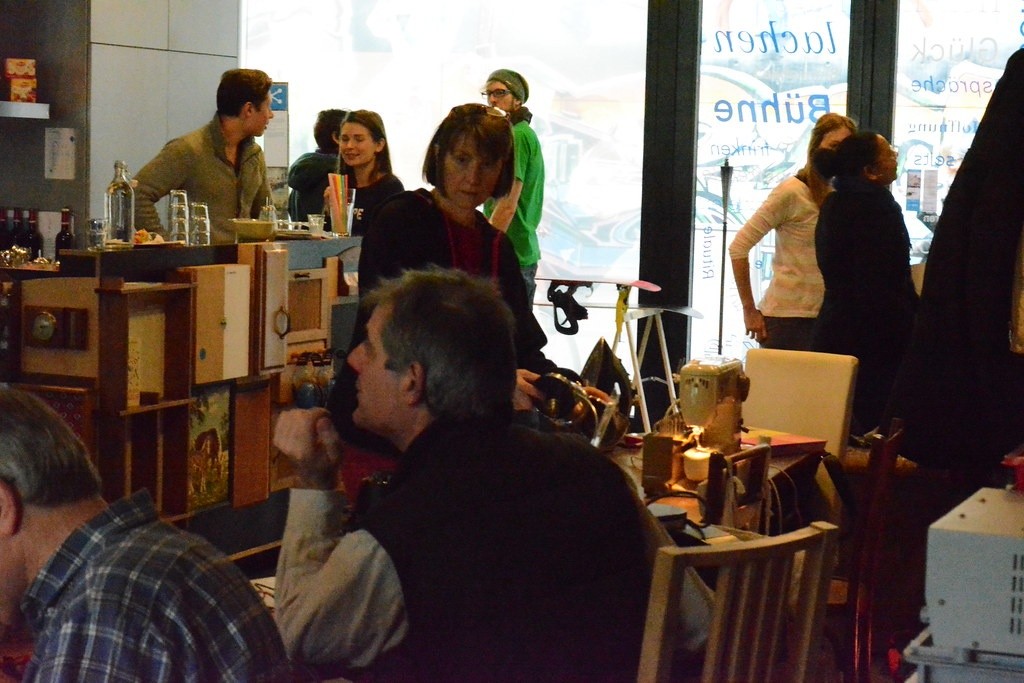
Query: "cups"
307 214 325 236
277 211 291 232
166 189 211 247
85 217 105 253
259 204 278 238
328 187 356 237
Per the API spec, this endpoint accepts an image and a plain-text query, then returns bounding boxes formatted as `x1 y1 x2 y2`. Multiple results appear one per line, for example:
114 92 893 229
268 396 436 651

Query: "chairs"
826 414 905 683
635 519 845 683
742 347 860 466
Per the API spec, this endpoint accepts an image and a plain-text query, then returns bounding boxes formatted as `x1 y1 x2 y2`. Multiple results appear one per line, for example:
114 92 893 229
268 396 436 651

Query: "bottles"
9 207 26 252
25 209 44 261
1 206 11 253
54 207 74 262
103 160 135 250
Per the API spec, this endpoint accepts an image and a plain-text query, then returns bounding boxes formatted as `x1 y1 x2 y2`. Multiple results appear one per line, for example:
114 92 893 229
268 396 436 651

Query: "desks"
603 427 822 522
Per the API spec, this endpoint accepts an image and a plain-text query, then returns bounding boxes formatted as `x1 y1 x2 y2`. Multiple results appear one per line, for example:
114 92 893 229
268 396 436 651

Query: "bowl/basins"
231 218 280 241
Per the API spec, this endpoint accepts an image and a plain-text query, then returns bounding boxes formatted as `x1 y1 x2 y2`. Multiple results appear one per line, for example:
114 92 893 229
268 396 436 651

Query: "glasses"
451 106 510 119
481 89 512 98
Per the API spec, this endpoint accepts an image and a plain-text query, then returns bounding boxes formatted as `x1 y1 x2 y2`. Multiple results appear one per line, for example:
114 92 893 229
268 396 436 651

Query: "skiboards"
531 275 704 343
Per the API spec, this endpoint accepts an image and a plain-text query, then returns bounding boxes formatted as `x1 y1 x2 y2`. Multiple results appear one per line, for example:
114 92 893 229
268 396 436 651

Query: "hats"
487 69 529 104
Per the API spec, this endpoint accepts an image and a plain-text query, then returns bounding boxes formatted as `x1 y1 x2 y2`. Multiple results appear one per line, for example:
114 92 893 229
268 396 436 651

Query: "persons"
321 107 590 513
1 383 303 681
319 110 402 234
260 266 717 683
730 113 858 351
284 106 353 234
122 64 279 244
807 130 919 449
480 70 544 313
